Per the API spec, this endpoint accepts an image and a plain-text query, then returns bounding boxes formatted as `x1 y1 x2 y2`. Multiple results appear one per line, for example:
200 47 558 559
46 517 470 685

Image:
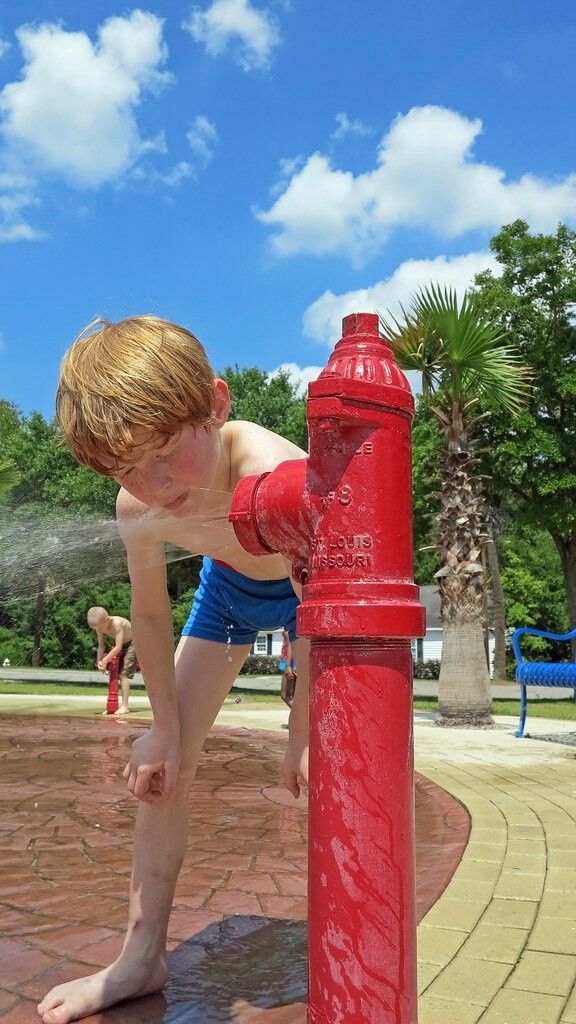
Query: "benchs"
512 628 576 738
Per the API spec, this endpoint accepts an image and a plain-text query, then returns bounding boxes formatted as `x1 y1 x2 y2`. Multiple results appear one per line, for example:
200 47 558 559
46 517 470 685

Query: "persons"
277 629 298 709
36 315 311 1024
87 607 137 714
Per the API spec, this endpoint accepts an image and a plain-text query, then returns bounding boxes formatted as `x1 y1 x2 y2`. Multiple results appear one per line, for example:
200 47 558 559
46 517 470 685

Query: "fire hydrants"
100 643 126 715
226 309 426 1024
